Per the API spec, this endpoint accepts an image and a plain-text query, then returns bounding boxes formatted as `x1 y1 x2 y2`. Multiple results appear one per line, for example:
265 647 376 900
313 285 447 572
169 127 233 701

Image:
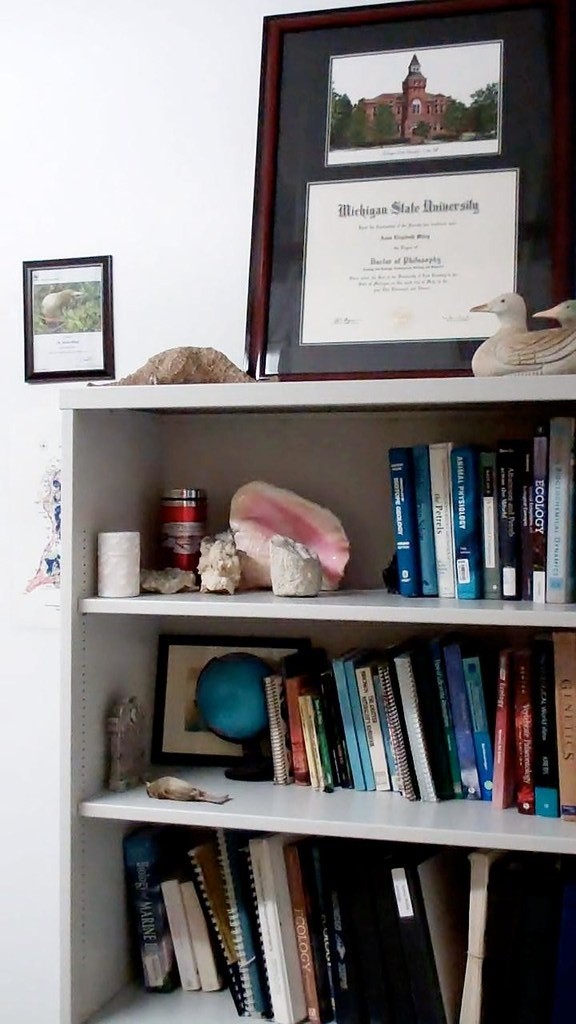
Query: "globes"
195 652 274 782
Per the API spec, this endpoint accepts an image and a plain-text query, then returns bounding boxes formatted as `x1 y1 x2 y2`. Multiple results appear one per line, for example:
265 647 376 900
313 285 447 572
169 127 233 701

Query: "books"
122 416 576 1024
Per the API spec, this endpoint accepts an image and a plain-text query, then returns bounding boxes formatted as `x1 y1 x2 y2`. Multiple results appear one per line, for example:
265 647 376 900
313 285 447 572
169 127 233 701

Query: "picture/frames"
246 0 576 380
23 255 115 383
151 634 312 767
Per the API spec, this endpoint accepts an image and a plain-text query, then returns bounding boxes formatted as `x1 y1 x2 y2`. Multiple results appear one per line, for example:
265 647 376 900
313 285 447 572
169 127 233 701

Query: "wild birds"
468 293 576 376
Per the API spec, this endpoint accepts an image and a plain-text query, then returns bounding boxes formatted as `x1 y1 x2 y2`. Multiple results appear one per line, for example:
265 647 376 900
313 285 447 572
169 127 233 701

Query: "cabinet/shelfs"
57 379 576 1024
